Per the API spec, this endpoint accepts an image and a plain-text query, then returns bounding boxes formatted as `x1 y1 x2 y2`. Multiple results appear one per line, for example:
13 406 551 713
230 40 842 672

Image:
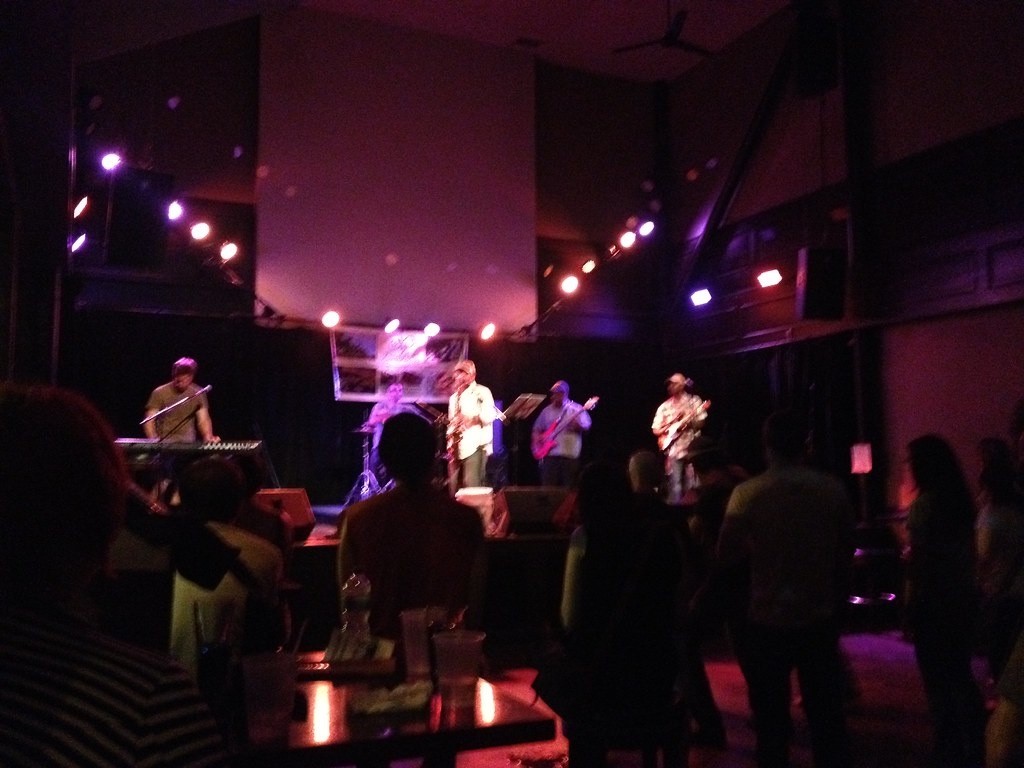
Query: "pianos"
114 438 263 458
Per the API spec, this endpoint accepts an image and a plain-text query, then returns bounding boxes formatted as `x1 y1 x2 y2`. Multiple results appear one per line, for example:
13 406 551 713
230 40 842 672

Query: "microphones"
189 384 213 402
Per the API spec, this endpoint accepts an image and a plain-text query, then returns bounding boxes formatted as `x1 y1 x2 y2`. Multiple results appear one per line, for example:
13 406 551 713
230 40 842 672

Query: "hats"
455 359 475 373
172 357 197 374
664 372 687 385
688 436 720 460
550 380 569 393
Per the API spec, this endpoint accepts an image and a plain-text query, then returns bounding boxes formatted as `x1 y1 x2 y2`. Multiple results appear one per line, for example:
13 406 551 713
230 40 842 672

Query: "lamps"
849 442 875 538
78 85 104 112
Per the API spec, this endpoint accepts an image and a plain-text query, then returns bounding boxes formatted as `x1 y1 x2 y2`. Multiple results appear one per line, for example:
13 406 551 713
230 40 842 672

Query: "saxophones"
443 385 463 471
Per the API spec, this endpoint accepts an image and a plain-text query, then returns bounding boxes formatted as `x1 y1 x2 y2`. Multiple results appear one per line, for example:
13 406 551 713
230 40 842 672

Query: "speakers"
104 165 175 267
492 487 567 536
252 487 317 543
796 247 849 320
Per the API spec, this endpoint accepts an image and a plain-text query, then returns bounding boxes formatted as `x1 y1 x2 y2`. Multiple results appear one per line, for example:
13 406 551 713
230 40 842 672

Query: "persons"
714 410 857 768
337 412 486 637
370 382 409 461
899 434 987 768
972 438 1024 682
448 360 494 492
681 436 744 548
169 456 284 667
561 451 726 768
533 381 591 484
144 357 214 443
651 374 708 492
0 381 228 768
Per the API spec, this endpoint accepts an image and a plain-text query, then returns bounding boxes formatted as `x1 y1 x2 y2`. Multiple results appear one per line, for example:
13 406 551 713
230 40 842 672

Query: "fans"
610 0 714 58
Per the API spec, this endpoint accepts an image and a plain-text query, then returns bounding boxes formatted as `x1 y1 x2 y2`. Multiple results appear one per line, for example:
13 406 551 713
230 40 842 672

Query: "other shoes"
689 725 728 749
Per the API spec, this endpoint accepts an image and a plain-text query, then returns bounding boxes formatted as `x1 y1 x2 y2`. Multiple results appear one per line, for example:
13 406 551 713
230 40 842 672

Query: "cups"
400 609 432 676
433 631 486 709
428 603 464 683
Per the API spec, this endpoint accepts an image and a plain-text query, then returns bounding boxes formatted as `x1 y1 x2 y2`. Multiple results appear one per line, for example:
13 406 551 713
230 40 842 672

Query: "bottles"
342 567 371 643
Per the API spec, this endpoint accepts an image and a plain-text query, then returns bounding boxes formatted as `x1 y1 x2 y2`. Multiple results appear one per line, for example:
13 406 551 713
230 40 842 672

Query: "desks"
286 651 558 768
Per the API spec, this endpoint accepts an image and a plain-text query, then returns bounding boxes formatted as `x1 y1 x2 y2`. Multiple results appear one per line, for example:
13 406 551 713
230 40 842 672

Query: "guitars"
530 395 599 460
657 400 712 453
